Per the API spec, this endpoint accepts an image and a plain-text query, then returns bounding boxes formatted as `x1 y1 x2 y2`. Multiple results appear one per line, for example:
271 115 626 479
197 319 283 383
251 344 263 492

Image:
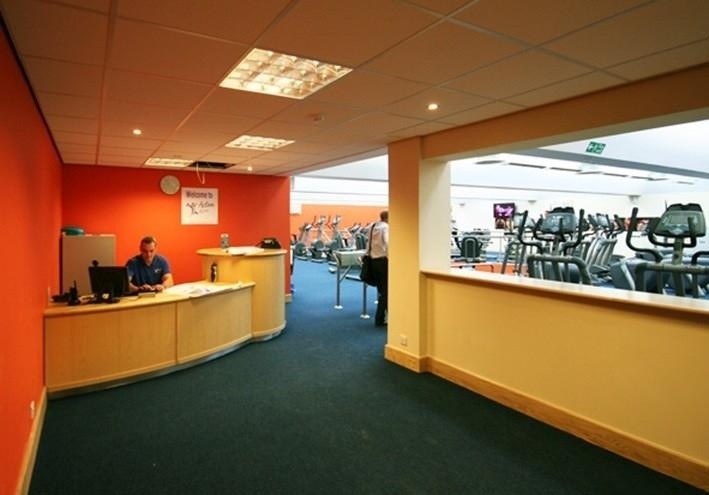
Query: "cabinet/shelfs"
60 232 116 299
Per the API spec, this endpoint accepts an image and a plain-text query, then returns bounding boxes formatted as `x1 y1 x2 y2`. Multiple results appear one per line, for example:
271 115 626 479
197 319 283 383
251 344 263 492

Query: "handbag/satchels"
359 257 381 286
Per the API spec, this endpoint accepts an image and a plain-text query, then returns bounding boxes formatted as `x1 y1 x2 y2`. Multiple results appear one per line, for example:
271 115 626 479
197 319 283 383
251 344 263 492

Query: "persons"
125 235 176 293
365 208 388 326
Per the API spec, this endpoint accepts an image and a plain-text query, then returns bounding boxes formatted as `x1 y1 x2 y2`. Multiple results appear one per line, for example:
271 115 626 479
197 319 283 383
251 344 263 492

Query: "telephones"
67 280 81 305
261 236 281 249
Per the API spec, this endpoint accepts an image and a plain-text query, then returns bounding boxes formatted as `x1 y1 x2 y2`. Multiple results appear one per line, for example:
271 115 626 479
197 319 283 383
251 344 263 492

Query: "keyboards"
123 289 158 296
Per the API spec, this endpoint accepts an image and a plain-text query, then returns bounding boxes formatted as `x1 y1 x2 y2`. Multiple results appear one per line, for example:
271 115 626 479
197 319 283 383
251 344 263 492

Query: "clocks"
160 174 181 196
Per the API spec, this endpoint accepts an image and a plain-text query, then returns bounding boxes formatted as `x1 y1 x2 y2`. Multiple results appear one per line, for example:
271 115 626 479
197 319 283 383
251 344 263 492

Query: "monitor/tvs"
88 265 129 304
493 203 515 217
